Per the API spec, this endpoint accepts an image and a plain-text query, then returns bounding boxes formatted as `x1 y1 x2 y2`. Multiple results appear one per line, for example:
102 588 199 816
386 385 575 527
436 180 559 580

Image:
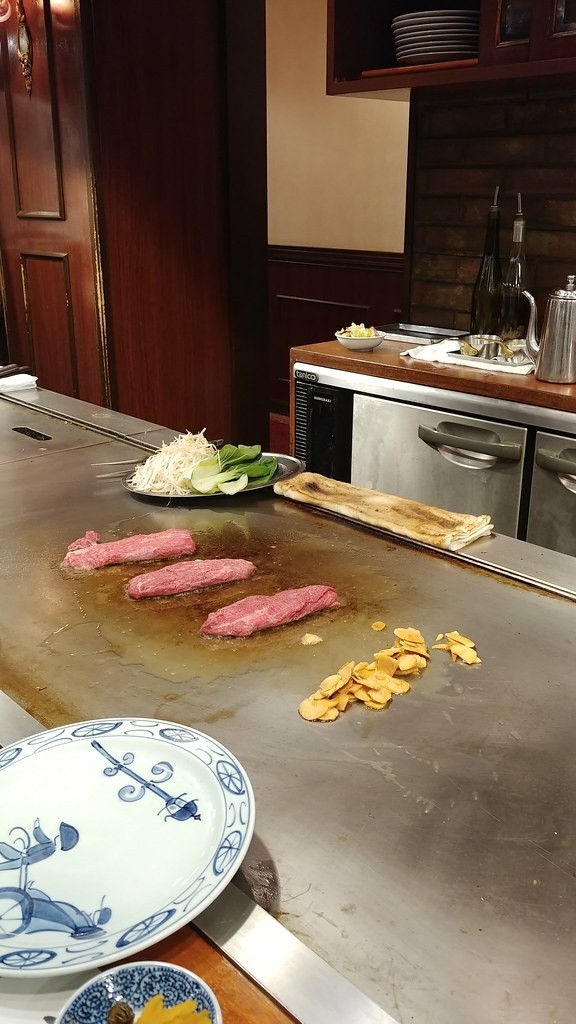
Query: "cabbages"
335 322 376 337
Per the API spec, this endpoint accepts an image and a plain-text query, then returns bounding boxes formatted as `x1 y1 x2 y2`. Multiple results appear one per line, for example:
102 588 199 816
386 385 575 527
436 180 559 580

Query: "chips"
298 621 482 721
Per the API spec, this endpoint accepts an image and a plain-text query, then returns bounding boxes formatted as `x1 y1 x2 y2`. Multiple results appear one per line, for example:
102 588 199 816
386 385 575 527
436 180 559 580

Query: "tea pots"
521 275 576 384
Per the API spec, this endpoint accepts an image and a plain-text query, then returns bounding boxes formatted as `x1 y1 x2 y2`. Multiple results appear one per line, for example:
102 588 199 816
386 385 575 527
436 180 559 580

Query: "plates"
0 718 257 975
391 10 480 64
122 452 306 498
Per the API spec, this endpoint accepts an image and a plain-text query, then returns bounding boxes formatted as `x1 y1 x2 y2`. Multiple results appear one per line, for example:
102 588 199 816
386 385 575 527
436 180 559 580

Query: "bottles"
497 192 527 340
470 182 502 335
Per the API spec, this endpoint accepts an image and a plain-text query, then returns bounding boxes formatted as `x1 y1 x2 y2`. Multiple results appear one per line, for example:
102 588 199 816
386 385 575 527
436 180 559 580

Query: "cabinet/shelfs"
0 0 241 450
290 341 576 558
326 0 576 103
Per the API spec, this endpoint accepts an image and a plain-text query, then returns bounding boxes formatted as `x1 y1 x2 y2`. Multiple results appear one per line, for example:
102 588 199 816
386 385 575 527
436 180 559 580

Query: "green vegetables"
183 444 278 495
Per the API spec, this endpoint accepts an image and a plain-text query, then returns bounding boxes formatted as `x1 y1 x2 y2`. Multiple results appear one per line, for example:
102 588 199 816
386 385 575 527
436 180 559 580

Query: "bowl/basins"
502 338 535 364
458 335 500 360
52 961 223 1024
334 330 386 351
